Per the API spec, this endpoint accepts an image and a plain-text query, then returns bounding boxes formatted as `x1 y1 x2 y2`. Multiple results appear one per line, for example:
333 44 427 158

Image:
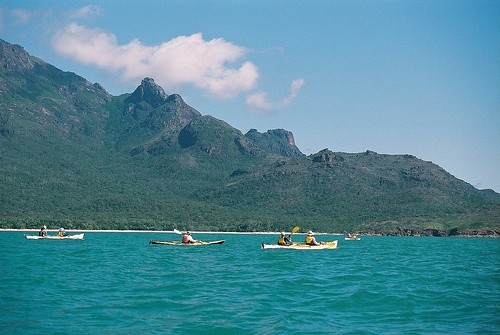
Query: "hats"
307 231 314 235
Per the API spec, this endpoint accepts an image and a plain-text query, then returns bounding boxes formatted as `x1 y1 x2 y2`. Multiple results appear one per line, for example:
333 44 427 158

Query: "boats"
344 237 361 240
148 239 226 246
261 239 339 250
25 233 85 240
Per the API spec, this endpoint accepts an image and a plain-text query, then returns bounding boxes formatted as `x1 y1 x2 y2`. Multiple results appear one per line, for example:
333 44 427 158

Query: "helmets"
60 228 63 232
281 232 285 236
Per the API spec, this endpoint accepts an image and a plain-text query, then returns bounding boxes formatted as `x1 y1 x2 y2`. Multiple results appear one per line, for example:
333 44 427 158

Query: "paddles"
317 241 335 248
285 226 299 239
174 228 208 245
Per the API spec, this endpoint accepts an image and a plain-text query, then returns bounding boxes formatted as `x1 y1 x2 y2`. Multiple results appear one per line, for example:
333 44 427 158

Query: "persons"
183 232 195 243
305 231 319 245
59 228 68 236
347 232 353 238
41 226 49 236
278 232 292 245
